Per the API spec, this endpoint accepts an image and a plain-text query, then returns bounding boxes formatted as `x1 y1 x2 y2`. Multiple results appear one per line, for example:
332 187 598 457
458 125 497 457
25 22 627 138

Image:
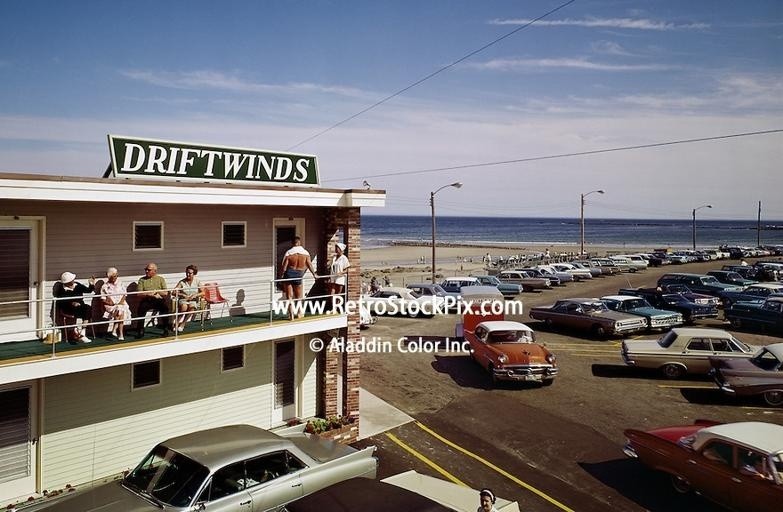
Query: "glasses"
186 271 196 274
146 269 155 272
113 274 120 278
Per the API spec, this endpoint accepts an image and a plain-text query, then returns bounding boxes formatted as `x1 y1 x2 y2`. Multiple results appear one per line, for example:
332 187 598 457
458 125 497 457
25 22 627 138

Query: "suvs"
657 273 743 296
706 271 759 286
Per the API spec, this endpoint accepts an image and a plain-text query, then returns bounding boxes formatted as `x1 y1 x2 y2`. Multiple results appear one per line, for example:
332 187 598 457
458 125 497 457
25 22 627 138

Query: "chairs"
205 282 232 323
136 291 171 327
172 298 204 327
62 313 95 345
101 301 127 335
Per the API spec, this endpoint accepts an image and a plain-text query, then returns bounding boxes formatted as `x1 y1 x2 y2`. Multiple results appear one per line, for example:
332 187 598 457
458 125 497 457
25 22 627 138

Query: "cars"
577 261 602 276
471 276 523 295
528 298 648 339
463 321 558 386
668 245 782 263
569 262 592 279
363 287 444 314
724 297 783 330
278 470 520 512
621 328 775 379
623 419 783 512
404 284 462 309
719 284 783 307
600 295 686 331
441 277 483 296
496 271 551 292
550 263 592 282
618 284 723 322
640 254 662 267
635 284 719 321
536 266 573 281
515 269 559 282
35 424 377 512
708 343 783 407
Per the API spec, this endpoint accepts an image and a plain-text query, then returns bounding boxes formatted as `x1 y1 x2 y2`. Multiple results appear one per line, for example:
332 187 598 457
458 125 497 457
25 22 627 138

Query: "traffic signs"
580 190 604 255
429 183 463 285
693 205 712 251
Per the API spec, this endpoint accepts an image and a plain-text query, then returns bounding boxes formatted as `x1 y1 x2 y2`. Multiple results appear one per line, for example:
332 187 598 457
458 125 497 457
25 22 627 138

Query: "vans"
611 258 639 272
616 255 647 270
755 263 783 274
590 258 620 274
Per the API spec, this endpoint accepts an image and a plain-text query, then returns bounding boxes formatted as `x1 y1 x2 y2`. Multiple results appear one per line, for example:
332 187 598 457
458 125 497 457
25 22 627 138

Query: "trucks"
455 286 504 340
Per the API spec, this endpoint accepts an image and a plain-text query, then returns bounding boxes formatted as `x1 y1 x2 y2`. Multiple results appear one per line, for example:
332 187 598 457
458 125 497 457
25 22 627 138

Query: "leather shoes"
67 327 91 343
135 331 146 339
165 328 171 337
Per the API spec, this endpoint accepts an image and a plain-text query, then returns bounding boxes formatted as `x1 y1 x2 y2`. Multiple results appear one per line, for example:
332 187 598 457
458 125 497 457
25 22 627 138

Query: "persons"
739 258 748 266
51 271 95 343
133 264 173 338
281 237 317 317
171 265 204 331
101 268 133 341
477 487 499 512
361 273 392 296
328 242 350 312
477 248 590 264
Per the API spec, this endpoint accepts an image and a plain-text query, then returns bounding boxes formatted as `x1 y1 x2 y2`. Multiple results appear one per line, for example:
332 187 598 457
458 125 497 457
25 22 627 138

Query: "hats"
59 272 78 284
336 243 346 252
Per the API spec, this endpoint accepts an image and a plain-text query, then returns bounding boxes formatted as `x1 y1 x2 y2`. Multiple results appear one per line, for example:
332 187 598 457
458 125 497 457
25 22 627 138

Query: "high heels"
173 325 185 332
112 332 125 342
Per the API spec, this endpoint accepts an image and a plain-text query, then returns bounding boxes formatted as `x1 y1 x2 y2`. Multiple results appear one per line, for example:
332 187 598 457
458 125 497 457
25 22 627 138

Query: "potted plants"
302 414 350 436
286 417 302 427
